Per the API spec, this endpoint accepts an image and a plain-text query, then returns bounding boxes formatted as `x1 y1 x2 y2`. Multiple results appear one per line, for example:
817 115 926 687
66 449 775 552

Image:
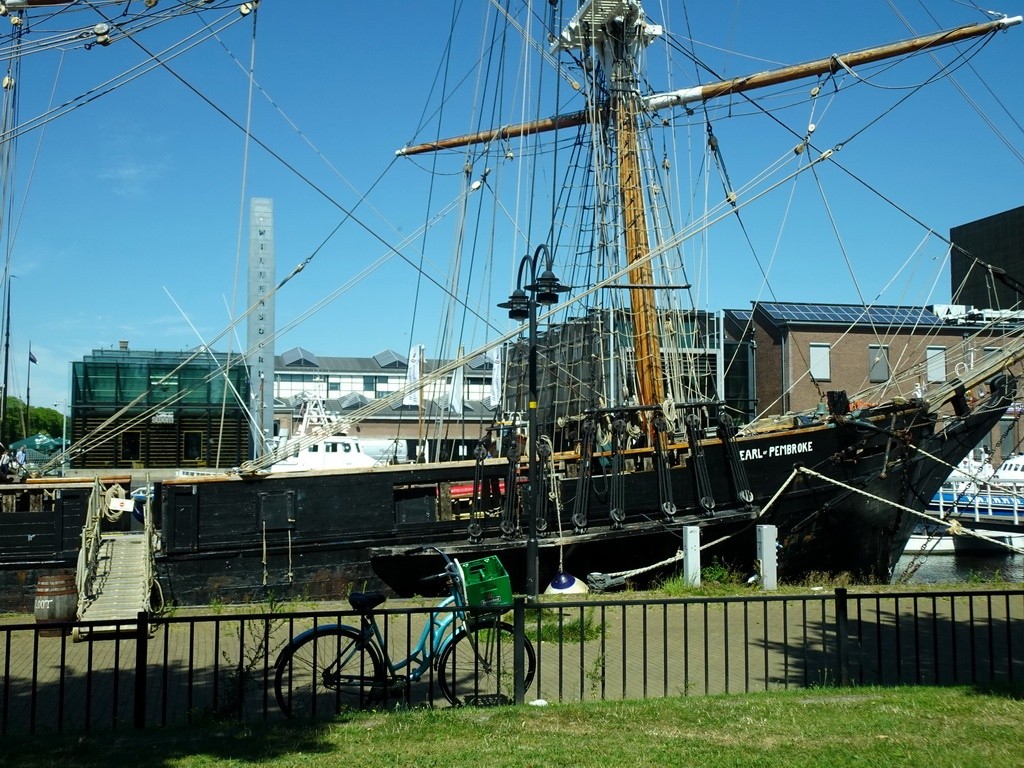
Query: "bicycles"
271 547 538 727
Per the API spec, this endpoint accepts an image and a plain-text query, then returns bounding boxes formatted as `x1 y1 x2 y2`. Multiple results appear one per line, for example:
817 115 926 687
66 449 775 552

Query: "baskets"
453 555 512 623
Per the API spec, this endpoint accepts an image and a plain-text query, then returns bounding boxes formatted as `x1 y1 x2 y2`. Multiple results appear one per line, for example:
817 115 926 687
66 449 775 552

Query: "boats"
912 441 1024 541
0 388 571 615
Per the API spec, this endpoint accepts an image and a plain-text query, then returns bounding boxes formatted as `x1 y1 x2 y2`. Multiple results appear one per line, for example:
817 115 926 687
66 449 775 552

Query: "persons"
0 446 26 475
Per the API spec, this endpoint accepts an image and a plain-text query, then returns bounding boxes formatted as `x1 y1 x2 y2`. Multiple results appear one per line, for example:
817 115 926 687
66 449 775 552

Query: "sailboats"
354 0 1024 597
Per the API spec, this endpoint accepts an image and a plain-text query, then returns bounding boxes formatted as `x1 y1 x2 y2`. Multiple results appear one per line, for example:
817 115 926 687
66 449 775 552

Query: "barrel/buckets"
33 575 77 638
